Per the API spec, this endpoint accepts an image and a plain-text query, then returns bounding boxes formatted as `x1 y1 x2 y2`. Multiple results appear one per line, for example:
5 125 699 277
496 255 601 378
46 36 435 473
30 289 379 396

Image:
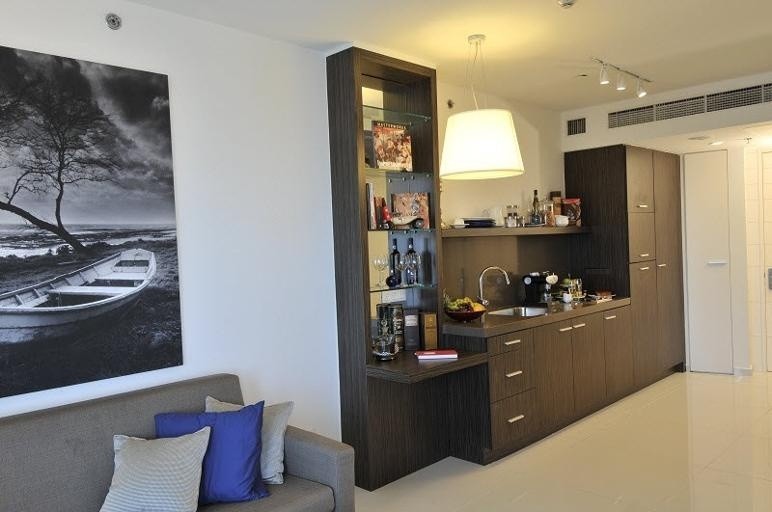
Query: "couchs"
0 371 356 511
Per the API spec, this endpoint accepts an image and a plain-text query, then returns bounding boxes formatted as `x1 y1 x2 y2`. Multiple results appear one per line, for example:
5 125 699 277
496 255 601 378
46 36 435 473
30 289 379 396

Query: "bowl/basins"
443 308 488 323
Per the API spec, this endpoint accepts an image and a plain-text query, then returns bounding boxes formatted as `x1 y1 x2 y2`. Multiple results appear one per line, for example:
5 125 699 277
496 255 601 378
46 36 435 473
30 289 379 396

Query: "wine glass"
372 254 422 289
568 278 583 307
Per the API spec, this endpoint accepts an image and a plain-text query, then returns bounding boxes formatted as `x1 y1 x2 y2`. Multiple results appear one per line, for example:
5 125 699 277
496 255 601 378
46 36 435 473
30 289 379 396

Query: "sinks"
487 304 548 318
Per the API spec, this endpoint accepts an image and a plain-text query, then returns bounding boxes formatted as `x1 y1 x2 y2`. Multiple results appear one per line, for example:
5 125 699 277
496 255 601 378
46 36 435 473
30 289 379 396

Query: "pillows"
205 390 295 486
152 399 270 505
100 427 211 511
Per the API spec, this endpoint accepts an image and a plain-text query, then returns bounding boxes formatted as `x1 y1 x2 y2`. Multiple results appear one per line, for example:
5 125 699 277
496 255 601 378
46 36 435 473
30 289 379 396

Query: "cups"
556 215 570 227
563 293 572 301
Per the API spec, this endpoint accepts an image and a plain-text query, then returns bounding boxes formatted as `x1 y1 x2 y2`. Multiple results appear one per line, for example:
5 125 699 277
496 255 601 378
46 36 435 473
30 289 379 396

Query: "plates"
561 298 574 303
588 294 617 300
449 224 470 229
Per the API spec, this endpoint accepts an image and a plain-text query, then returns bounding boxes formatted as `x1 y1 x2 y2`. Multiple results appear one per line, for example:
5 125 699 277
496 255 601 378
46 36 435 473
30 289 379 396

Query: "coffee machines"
522 271 549 307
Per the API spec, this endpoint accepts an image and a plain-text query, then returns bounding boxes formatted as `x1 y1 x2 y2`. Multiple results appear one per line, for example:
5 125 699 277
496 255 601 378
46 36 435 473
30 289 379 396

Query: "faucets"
476 266 510 305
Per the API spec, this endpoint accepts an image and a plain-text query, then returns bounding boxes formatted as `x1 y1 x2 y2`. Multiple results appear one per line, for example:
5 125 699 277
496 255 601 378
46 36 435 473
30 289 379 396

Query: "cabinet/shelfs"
563 146 656 213
534 310 607 444
442 328 529 466
577 259 665 396
602 304 635 409
563 213 656 270
654 150 686 381
323 45 489 492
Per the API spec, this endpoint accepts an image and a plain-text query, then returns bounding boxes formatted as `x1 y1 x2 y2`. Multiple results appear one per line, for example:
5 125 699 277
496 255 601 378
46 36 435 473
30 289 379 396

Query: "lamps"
435 33 529 184
589 56 653 99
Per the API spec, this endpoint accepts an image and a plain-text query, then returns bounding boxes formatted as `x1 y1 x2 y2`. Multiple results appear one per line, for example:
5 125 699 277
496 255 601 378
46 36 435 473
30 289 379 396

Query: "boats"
1 247 158 325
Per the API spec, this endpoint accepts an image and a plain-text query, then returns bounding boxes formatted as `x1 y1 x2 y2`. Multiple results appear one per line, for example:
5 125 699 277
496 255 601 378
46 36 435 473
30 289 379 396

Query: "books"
416 349 458 360
391 192 430 229
365 182 377 231
371 120 414 173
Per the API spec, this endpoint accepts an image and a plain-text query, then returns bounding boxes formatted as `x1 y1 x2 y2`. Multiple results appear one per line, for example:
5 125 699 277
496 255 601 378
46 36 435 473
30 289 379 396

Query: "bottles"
504 199 555 227
388 237 403 287
532 189 542 227
404 236 420 286
